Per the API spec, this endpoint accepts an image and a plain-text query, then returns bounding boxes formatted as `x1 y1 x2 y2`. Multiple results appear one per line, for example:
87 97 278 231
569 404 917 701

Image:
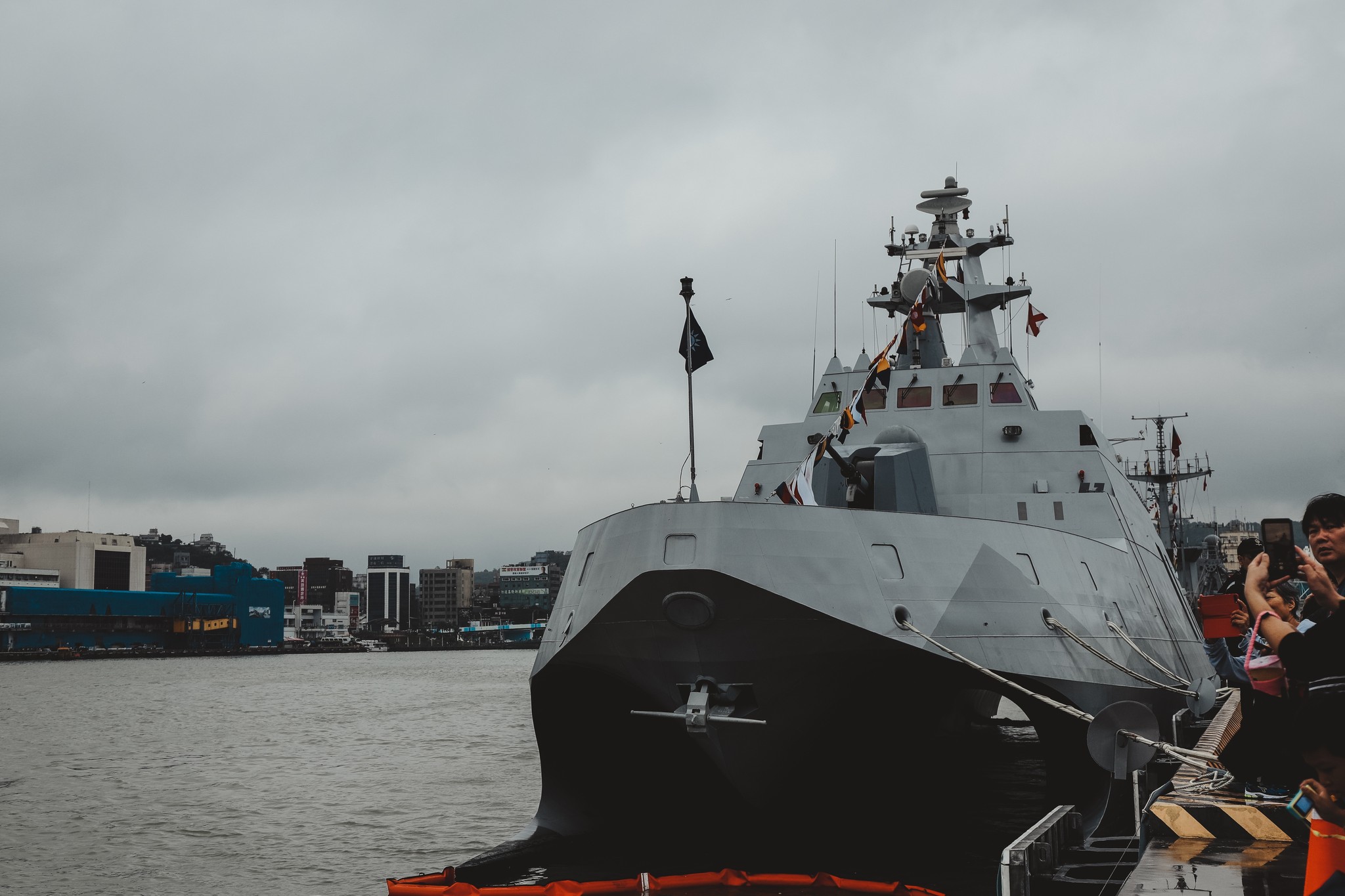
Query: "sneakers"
1244 781 1289 799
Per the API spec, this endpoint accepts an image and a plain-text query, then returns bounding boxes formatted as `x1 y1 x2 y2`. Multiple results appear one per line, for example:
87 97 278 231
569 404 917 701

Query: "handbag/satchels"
1244 611 1289 696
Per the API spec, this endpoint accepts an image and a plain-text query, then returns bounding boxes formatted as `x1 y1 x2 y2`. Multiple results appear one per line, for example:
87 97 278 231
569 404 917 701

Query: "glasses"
1243 554 1257 560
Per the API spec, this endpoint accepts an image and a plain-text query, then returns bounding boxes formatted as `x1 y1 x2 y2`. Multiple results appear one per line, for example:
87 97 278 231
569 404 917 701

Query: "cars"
504 639 513 643
492 625 498 626
507 624 513 625
467 640 475 643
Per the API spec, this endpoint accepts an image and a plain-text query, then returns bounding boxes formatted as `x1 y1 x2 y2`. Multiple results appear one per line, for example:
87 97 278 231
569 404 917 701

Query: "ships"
530 170 1234 857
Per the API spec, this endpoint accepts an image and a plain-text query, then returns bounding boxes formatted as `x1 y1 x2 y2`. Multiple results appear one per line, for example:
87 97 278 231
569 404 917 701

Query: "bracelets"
1261 613 1269 619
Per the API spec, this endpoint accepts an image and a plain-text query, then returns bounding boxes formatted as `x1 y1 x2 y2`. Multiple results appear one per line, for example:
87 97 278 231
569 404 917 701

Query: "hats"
1237 538 1264 553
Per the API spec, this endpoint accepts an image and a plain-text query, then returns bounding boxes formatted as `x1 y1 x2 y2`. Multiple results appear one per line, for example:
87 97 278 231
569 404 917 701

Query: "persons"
1196 494 1345 829
539 567 548 575
541 589 547 594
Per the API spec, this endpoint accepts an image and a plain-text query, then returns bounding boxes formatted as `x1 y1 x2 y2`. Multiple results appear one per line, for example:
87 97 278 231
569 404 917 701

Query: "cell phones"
1201 594 1240 617
1261 518 1298 582
1287 785 1317 820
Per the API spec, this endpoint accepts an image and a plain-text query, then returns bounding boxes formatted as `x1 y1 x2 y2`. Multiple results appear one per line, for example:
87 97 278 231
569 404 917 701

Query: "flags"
897 250 948 355
1026 302 1048 337
680 307 714 374
1203 475 1207 491
1143 459 1179 579
1171 425 1182 462
778 333 898 506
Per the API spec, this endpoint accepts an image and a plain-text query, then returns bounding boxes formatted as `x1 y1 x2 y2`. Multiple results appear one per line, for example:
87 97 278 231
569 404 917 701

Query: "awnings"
283 637 305 641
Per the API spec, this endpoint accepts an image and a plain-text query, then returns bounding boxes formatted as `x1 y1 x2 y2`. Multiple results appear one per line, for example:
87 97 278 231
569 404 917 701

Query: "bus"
321 638 349 645
534 619 549 624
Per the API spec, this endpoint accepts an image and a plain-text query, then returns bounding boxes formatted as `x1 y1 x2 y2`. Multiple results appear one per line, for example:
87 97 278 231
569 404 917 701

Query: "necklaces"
1289 616 1294 622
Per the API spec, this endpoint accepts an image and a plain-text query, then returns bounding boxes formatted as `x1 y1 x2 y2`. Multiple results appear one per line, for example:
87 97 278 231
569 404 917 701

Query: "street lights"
532 610 536 624
428 621 432 629
479 637 481 646
479 612 482 627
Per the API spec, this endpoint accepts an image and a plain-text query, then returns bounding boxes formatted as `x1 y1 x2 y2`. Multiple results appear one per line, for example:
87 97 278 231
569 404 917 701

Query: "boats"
361 643 388 652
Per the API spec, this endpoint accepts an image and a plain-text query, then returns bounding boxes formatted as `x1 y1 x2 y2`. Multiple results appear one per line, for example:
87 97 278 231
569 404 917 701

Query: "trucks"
302 641 316 647
